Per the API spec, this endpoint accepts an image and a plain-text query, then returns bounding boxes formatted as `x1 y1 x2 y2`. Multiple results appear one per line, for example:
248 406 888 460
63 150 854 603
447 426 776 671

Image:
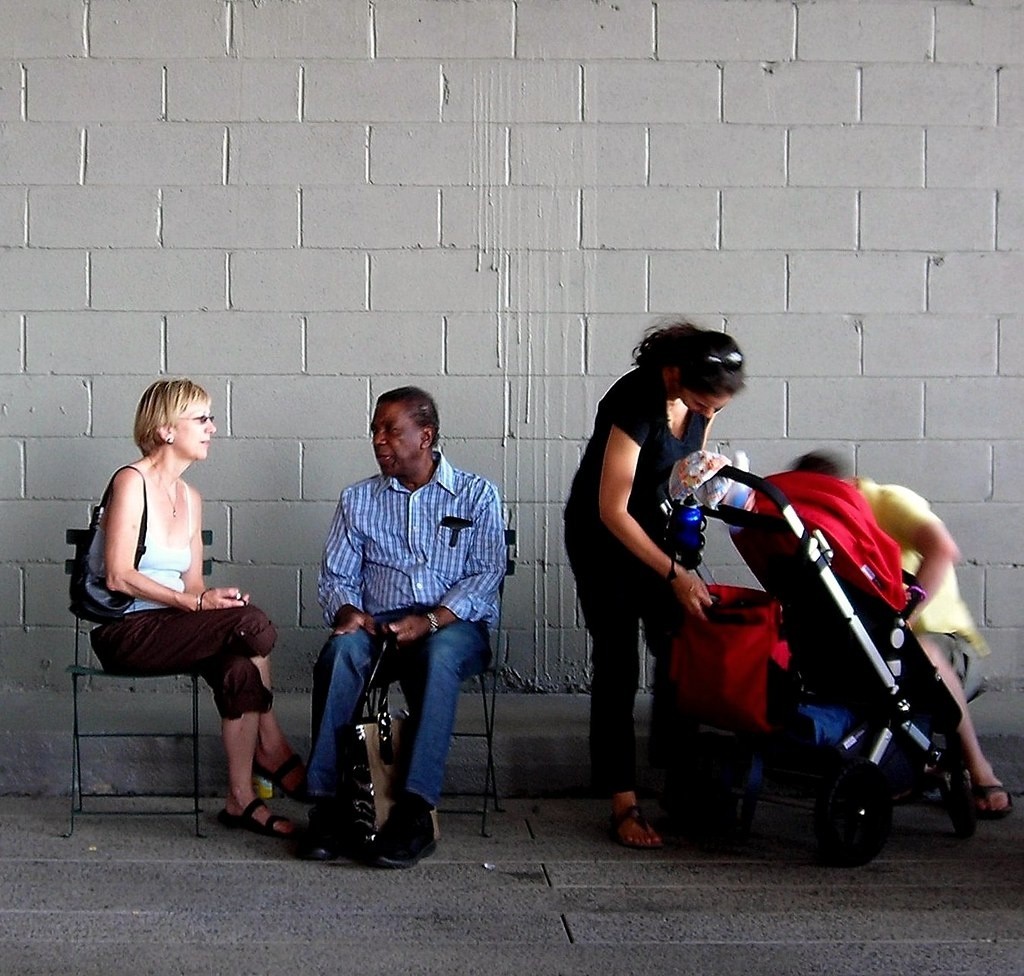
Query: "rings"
689 585 694 591
241 600 246 606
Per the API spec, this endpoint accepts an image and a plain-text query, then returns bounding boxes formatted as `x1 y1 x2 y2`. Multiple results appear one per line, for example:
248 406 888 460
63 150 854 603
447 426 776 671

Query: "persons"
87 379 319 832
563 325 743 849
791 449 1014 817
289 387 507 869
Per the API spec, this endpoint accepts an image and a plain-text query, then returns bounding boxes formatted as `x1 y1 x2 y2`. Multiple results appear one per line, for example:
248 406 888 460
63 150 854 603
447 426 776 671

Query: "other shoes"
370 798 436 869
296 802 351 860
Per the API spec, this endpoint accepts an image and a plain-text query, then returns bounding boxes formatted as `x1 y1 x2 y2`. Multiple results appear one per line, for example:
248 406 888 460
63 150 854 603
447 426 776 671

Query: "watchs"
426 613 438 635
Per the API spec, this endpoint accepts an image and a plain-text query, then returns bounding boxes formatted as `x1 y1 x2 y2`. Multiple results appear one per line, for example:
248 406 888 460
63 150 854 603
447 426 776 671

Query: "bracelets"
906 620 912 631
196 593 201 612
666 561 677 583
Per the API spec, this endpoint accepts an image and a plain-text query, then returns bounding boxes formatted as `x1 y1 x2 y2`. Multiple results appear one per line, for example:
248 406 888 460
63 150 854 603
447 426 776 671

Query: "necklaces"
148 455 178 517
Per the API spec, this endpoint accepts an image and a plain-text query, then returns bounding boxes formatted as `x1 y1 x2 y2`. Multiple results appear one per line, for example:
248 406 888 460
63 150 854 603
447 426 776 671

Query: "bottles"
678 496 702 557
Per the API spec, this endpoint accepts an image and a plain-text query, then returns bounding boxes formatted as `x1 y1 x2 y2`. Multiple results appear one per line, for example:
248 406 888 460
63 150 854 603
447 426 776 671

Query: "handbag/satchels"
661 583 790 740
334 629 442 845
68 465 149 624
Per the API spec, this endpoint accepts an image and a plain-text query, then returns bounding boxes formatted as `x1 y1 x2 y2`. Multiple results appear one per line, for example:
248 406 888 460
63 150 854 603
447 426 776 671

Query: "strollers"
653 464 982 868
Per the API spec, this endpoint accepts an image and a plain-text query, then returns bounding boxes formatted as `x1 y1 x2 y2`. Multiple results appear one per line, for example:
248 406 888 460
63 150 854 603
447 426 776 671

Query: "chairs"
376 530 518 837
60 528 214 838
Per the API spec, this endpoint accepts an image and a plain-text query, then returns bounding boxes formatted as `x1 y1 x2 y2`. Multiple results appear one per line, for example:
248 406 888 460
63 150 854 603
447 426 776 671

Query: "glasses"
180 415 215 424
694 351 746 378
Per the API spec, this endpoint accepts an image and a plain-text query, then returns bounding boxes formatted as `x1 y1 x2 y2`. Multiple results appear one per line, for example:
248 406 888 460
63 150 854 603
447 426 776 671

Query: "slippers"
608 805 665 849
251 753 315 805
972 783 1013 820
216 798 303 838
